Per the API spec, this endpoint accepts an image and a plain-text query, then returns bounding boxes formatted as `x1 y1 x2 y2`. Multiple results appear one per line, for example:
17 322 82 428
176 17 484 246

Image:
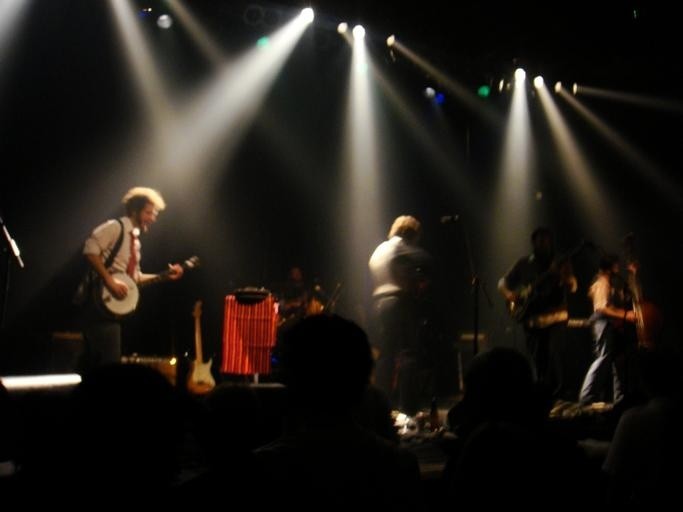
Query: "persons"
366 215 433 416
1 313 682 511
72 186 183 373
497 226 652 410
277 265 335 321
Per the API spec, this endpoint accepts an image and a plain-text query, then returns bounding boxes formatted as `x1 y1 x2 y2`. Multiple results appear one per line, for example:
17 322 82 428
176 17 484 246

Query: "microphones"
440 215 461 224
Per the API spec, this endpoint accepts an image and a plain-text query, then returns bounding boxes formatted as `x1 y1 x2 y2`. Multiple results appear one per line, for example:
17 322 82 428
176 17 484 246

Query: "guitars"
188 300 214 395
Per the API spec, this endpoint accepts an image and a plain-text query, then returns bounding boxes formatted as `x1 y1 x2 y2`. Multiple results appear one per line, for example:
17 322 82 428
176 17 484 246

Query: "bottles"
429 396 440 431
